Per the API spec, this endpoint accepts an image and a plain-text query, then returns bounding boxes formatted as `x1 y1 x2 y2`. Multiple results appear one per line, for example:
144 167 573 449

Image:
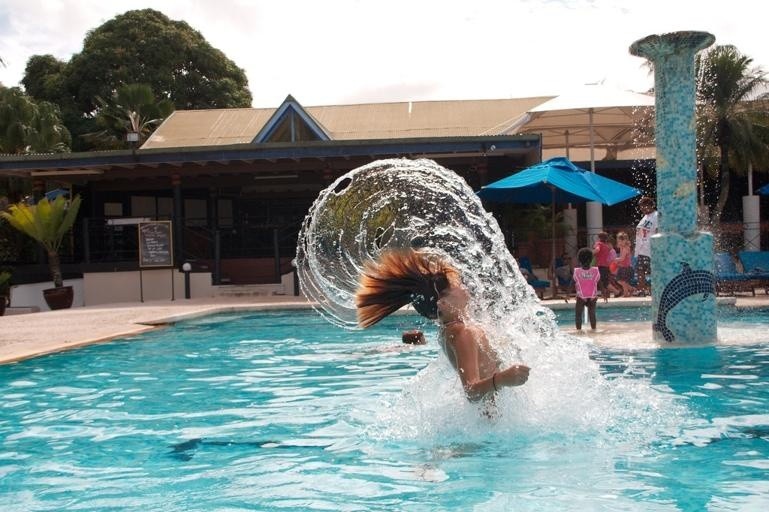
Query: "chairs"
513 248 769 302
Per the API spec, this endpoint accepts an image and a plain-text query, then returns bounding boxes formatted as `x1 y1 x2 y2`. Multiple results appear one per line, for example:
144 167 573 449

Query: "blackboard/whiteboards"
138 220 173 268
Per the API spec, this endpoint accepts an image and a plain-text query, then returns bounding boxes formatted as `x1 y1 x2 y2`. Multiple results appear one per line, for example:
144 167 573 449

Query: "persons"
514 256 547 289
592 232 619 297
635 197 658 296
565 247 601 331
354 241 532 471
555 253 574 292
402 330 428 348
607 237 617 284
613 231 636 297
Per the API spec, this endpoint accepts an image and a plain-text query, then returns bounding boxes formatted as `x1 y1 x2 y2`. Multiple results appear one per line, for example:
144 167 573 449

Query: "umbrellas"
468 157 643 296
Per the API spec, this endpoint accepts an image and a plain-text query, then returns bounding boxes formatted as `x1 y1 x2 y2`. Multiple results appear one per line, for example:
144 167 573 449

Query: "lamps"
126 131 139 161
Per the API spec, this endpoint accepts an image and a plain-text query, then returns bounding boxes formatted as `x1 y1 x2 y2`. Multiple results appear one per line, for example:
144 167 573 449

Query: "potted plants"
0 189 87 310
1 271 13 316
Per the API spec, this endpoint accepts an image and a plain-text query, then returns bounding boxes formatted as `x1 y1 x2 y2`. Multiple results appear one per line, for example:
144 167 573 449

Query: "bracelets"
492 373 498 391
